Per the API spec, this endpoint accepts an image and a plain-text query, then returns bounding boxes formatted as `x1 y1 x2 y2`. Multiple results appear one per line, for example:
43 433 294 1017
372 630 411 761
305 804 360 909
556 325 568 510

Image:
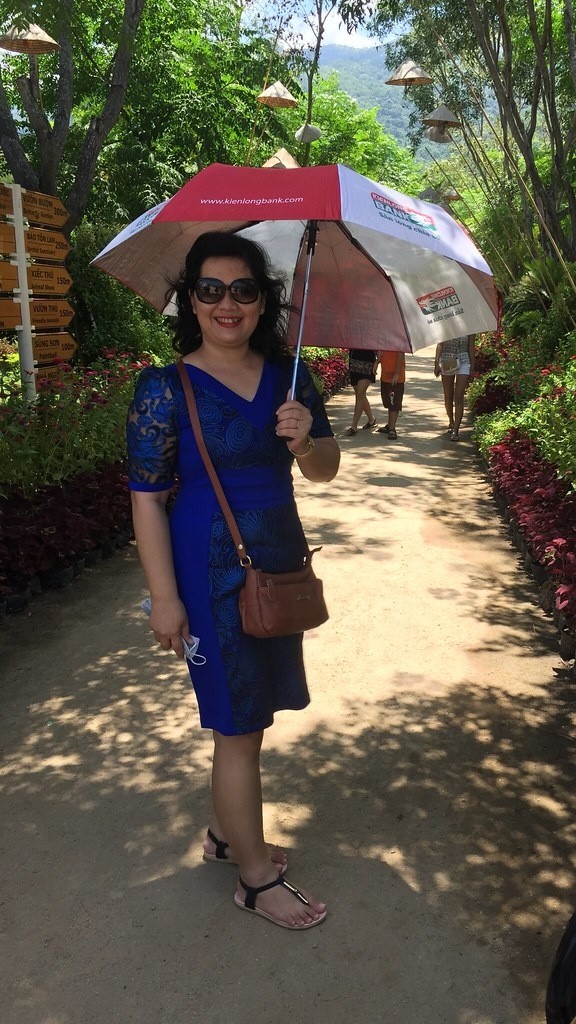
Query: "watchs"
288 435 315 458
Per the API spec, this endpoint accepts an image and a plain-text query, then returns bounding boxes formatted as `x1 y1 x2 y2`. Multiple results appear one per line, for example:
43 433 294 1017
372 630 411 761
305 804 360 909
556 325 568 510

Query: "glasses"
196 277 261 304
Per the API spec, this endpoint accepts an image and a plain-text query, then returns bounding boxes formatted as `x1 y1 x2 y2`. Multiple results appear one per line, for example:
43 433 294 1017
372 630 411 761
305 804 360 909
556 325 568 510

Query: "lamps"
255 56 464 216
0 19 61 55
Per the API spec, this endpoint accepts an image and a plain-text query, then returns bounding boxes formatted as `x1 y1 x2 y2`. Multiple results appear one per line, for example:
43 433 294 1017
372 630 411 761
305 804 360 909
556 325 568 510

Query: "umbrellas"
89 162 500 401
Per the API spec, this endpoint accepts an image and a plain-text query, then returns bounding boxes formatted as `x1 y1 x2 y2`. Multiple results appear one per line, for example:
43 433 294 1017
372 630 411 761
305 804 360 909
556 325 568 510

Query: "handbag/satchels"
442 357 460 373
239 559 329 638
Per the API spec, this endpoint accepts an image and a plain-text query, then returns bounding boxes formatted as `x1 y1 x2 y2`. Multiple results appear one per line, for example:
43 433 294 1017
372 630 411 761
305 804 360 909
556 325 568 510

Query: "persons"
434 334 477 441
372 350 406 440
343 348 377 437
126 231 341 930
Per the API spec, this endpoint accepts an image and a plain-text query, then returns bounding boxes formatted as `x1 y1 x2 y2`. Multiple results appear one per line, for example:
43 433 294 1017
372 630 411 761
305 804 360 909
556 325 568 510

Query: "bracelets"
435 359 439 363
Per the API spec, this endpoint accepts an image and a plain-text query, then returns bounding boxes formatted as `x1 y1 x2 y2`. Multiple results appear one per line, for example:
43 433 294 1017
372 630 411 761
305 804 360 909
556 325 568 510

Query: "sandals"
205 829 288 874
234 874 327 929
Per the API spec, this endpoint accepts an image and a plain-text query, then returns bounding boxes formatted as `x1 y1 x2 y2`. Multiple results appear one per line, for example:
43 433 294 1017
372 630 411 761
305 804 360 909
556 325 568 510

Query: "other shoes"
388 429 397 440
362 418 376 430
451 434 459 441
377 425 389 433
448 424 454 433
345 428 358 436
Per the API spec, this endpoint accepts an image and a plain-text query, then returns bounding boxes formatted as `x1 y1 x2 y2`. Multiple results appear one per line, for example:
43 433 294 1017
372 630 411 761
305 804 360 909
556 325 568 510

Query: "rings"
295 420 300 428
